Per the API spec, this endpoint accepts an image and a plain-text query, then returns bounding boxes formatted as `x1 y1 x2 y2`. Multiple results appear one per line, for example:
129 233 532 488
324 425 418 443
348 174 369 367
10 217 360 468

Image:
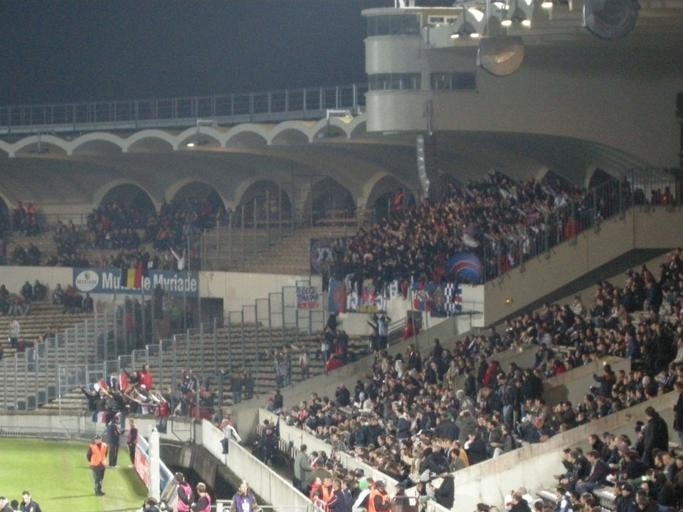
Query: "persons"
9 500 22 512
19 489 41 512
0 495 11 511
305 150 681 512
77 342 304 512
0 190 212 348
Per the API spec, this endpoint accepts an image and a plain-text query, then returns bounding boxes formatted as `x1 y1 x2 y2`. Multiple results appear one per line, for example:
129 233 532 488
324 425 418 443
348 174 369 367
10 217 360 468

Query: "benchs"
4 221 355 280
0 295 340 427
513 437 683 512
